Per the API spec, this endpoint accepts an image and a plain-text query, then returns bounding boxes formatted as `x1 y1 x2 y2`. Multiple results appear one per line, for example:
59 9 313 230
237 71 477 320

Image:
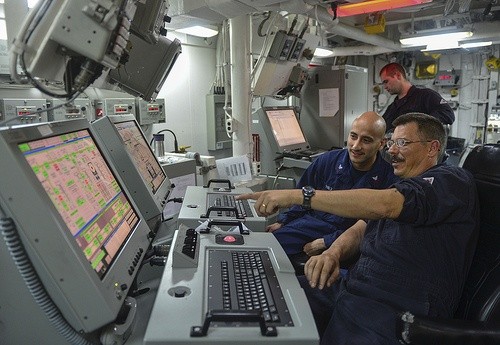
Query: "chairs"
396 142 500 345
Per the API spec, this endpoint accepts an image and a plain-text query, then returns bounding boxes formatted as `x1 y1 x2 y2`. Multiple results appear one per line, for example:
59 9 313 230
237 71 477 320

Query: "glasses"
387 139 440 151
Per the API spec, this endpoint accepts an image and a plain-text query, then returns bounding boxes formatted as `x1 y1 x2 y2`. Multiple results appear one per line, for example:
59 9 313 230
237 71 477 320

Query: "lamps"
420 38 495 53
174 19 218 39
398 29 475 45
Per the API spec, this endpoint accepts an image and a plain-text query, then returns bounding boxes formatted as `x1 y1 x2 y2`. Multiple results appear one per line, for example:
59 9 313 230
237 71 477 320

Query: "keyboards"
292 149 326 158
207 193 254 220
207 245 294 328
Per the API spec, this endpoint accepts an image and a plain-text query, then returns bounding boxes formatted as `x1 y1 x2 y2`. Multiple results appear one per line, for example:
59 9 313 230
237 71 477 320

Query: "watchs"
302 185 314 209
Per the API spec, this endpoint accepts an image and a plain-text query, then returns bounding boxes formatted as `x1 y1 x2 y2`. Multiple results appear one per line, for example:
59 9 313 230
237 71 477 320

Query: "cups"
153 134 164 157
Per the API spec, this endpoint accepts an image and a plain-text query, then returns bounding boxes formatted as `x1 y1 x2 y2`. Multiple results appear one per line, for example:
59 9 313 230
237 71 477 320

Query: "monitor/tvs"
92 114 174 220
0 117 154 333
258 105 311 159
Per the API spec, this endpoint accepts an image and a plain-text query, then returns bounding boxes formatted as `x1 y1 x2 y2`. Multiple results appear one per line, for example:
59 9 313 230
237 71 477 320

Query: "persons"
267 110 402 260
235 113 479 345
379 63 456 135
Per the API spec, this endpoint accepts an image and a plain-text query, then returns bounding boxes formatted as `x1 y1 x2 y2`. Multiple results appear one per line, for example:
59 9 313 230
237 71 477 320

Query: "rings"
262 204 266 207
